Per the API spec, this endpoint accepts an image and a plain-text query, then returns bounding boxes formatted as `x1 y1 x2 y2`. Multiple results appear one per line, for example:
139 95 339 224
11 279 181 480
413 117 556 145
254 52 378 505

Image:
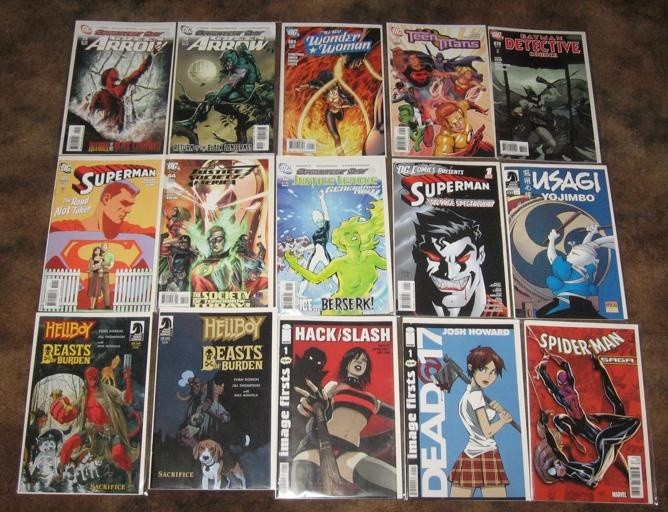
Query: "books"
15 19 654 501
